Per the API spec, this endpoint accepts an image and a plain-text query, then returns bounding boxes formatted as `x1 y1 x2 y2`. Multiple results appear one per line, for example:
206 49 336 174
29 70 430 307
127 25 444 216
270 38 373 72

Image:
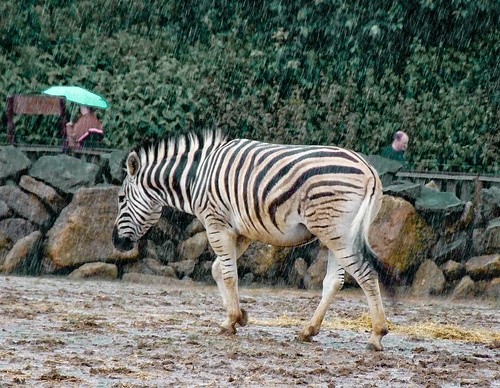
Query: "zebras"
111 124 389 351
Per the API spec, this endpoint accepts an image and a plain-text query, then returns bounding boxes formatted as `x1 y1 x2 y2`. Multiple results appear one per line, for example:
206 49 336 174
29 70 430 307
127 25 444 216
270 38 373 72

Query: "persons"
66 106 104 149
381 131 409 162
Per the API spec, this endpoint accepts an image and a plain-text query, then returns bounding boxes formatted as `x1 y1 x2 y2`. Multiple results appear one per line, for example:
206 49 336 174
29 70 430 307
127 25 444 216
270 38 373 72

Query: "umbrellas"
41 85 107 124
